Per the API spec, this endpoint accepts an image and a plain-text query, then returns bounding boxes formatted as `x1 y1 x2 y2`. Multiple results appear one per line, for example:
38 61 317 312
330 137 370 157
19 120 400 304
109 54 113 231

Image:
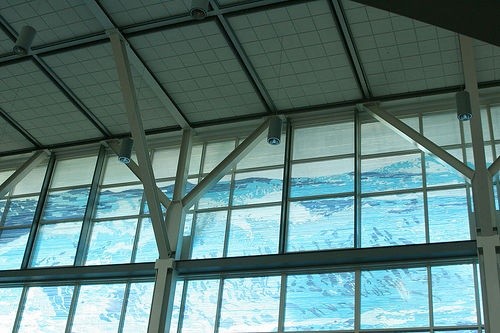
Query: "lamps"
118 59 146 163
454 32 473 120
267 37 283 145
189 0 208 18
13 24 36 55
